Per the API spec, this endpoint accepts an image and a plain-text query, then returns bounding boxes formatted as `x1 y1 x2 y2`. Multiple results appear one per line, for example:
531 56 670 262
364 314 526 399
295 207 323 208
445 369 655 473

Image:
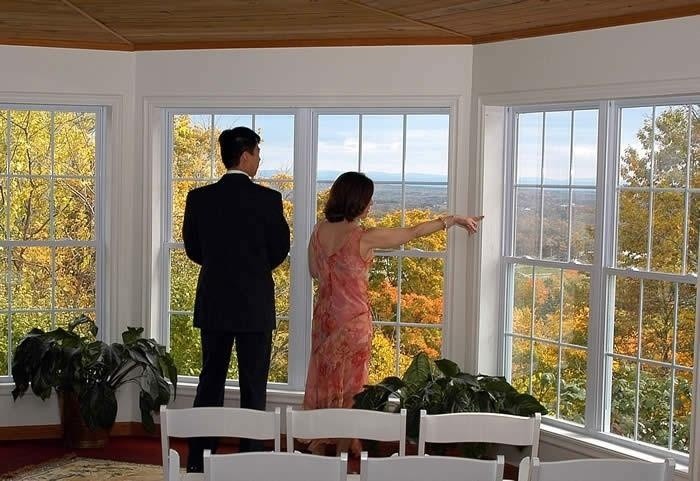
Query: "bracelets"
439 216 447 231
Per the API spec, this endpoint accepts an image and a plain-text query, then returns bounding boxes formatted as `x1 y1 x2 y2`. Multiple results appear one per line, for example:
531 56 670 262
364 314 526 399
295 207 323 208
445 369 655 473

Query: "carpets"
0 451 188 481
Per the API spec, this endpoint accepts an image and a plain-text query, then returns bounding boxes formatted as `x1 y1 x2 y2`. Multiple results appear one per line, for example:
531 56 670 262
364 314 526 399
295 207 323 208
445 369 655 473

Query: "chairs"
417 409 542 458
162 404 282 479
202 449 672 479
286 405 408 458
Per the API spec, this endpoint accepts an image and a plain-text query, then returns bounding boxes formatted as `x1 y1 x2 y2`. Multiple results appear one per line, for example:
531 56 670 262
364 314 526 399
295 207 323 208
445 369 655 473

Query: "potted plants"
352 352 548 459
11 314 177 451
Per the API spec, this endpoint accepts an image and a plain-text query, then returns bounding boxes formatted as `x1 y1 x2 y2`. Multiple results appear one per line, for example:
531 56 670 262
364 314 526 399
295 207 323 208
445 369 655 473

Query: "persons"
183 126 291 474
295 170 485 456
